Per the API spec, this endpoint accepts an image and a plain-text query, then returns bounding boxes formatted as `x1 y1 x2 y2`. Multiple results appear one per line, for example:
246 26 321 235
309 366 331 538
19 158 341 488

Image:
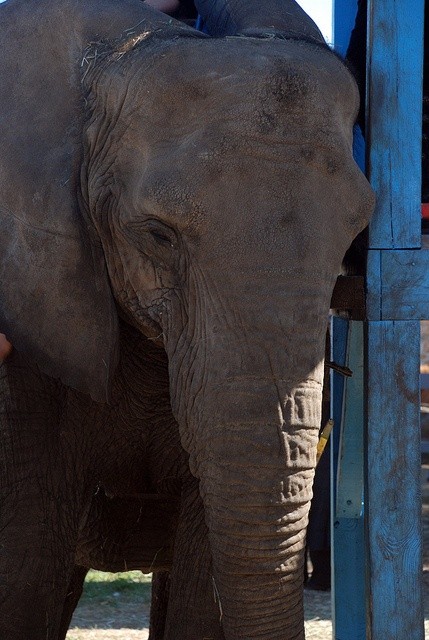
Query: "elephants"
0 0 376 640
